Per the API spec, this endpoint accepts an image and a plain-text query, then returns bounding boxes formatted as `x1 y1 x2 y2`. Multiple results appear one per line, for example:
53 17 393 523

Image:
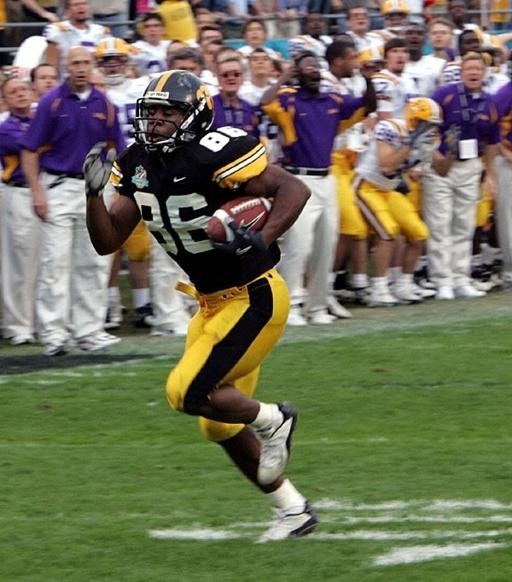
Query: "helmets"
406 98 443 132
382 0 410 14
129 70 214 154
93 39 132 65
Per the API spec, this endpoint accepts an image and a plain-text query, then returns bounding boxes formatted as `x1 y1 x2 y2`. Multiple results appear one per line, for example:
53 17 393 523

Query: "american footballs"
207 195 274 242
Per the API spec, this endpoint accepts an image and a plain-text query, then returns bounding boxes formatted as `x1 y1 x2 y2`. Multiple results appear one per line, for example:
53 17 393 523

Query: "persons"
1 0 512 361
83 69 317 545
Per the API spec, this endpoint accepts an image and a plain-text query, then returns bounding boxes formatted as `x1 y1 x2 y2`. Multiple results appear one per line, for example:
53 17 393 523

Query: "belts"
46 169 85 181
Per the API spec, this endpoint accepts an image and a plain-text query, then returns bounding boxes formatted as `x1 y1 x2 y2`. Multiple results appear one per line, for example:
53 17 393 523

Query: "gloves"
83 141 116 196
209 219 264 255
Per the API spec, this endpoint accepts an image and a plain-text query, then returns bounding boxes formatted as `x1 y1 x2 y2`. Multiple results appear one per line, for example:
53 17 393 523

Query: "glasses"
224 72 239 78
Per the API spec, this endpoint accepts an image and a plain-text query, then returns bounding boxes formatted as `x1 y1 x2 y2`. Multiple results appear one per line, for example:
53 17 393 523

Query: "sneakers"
286 294 351 326
40 333 72 356
130 300 154 330
8 334 36 345
347 275 502 308
104 305 128 333
151 302 191 338
77 328 122 352
